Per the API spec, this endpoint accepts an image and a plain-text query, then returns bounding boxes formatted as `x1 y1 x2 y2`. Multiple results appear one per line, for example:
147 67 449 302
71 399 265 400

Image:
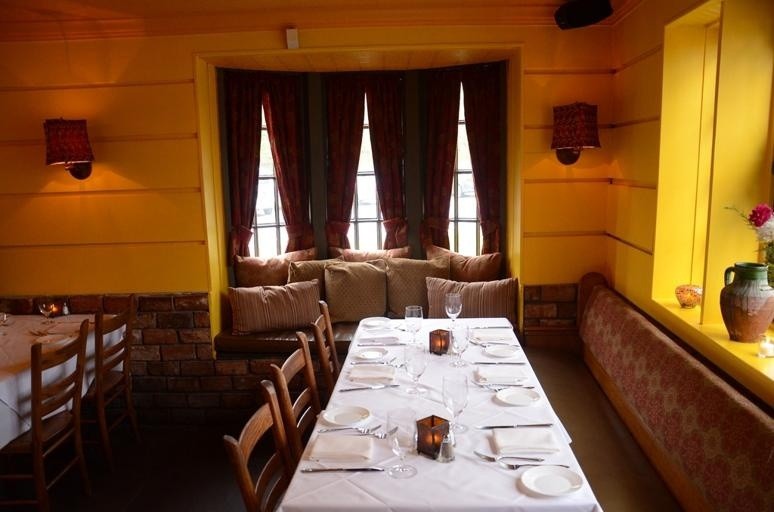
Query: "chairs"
2 294 137 509
218 298 339 512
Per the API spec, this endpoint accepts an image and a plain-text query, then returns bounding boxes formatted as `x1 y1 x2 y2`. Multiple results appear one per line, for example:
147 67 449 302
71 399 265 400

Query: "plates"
484 345 520 357
36 334 69 345
362 316 391 328
520 466 584 496
355 348 389 359
495 387 542 406
322 406 371 425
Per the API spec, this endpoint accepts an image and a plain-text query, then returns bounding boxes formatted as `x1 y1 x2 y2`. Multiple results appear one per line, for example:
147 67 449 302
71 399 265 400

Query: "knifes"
475 362 527 364
472 326 512 329
300 467 385 473
340 384 400 392
475 423 554 429
357 343 406 346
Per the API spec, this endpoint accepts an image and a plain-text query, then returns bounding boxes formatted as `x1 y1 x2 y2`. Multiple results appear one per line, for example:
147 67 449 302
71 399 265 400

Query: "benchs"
519 272 773 510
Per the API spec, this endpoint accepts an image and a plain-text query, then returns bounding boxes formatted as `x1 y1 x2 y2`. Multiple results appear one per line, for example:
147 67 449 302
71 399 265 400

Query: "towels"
307 319 562 460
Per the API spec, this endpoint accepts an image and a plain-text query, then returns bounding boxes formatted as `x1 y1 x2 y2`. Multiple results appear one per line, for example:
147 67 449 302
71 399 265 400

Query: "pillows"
227 241 518 340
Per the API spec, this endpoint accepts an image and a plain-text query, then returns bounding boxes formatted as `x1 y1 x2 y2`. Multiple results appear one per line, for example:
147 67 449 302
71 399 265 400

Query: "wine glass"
386 408 418 478
450 325 470 367
404 347 428 396
441 373 469 433
38 301 54 327
404 305 424 345
445 293 462 330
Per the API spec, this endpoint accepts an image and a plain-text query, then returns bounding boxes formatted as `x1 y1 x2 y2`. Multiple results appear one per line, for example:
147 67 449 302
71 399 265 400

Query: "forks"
496 459 570 470
345 427 399 439
473 450 545 462
317 424 382 433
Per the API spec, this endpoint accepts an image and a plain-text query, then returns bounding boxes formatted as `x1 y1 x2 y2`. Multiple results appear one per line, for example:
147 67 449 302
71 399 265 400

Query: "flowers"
723 203 773 286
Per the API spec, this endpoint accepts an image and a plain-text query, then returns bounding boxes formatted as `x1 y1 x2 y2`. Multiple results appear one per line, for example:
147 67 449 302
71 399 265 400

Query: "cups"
758 333 774 358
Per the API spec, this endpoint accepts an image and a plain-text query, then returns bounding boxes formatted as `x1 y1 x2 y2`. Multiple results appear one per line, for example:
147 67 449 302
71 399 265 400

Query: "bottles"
438 434 456 464
63 302 69 315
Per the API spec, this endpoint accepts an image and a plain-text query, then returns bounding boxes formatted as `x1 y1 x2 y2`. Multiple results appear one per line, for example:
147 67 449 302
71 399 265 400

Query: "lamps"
40 117 97 182
549 104 604 166
